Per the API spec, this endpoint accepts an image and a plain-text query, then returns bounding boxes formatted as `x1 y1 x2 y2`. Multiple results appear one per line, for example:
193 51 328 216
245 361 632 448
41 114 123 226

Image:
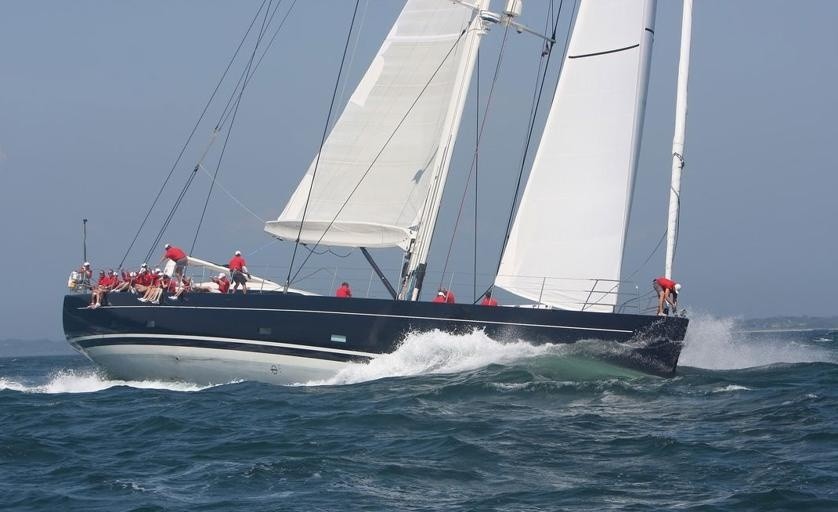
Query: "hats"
235 251 240 255
218 273 225 278
675 284 681 295
438 292 446 298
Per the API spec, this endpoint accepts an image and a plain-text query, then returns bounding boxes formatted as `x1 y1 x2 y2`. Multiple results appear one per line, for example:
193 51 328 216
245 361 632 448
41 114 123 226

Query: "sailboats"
61 1 692 387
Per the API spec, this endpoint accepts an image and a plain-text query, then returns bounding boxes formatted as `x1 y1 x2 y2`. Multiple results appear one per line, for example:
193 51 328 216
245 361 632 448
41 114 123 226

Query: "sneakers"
658 312 666 316
168 296 178 300
88 303 101 309
137 298 159 304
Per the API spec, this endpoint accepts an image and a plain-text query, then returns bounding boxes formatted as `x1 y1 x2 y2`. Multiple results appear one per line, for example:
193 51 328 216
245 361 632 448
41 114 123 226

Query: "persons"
481 293 497 306
336 283 352 298
443 289 455 303
229 250 250 294
653 277 681 316
209 273 230 294
433 292 446 302
79 244 193 309
